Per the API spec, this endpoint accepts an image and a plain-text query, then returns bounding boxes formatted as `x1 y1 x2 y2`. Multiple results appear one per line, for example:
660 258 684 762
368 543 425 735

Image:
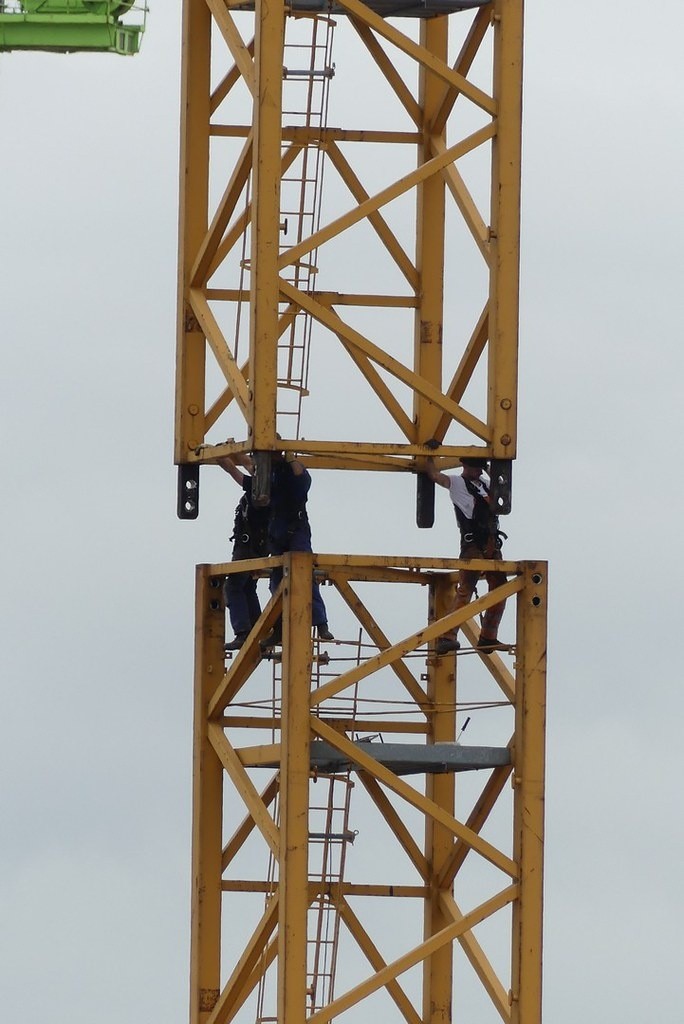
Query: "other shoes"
225 635 246 650
260 644 267 655
317 622 334 640
263 630 282 647
477 639 509 654
437 638 460 655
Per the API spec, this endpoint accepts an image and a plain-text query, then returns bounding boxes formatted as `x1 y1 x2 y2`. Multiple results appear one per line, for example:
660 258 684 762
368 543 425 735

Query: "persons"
226 432 334 646
424 440 513 655
196 444 272 650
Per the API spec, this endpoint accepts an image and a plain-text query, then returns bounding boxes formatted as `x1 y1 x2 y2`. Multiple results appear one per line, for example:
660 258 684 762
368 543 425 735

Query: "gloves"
424 439 442 450
195 443 213 456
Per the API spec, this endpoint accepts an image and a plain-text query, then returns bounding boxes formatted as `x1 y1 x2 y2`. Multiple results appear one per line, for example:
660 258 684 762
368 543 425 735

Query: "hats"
459 445 487 469
